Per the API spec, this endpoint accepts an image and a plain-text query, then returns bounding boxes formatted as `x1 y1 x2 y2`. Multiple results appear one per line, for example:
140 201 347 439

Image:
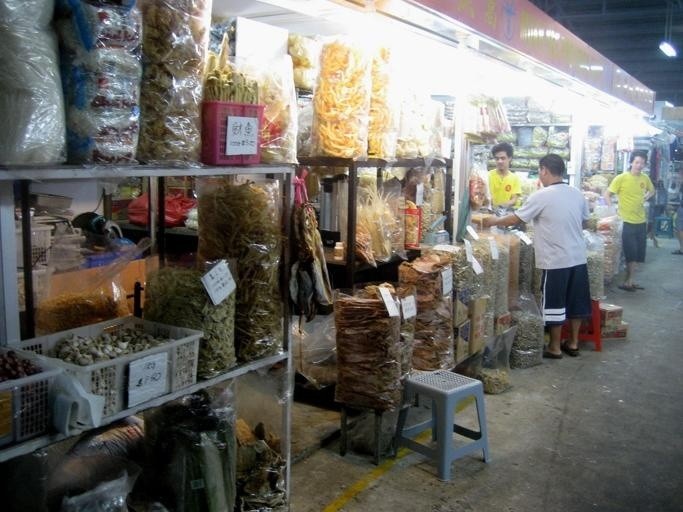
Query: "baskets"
17 265 54 312
198 101 266 166
4 314 205 429
51 227 81 264
15 221 54 269
0 346 64 451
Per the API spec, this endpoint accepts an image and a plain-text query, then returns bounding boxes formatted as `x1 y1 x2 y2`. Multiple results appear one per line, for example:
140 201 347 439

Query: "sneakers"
670 249 683 256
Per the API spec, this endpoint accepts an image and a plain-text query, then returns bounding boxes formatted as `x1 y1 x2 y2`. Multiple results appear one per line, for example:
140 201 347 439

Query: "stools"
388 367 494 483
558 298 603 353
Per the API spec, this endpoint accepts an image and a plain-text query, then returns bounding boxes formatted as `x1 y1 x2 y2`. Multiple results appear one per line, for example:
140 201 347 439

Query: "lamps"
657 2 678 57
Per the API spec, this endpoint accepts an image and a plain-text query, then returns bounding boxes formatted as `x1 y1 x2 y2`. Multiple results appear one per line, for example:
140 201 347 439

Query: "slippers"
618 285 636 292
542 343 562 359
561 339 579 358
632 284 645 289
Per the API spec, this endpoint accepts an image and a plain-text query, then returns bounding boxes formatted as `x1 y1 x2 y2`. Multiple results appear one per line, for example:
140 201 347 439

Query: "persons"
605 151 655 289
484 154 591 359
657 180 667 215
74 213 142 266
671 170 683 254
487 143 521 208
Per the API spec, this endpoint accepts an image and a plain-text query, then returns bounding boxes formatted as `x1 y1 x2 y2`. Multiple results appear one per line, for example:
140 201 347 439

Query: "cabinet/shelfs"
475 125 582 210
2 163 296 511
297 158 453 420
